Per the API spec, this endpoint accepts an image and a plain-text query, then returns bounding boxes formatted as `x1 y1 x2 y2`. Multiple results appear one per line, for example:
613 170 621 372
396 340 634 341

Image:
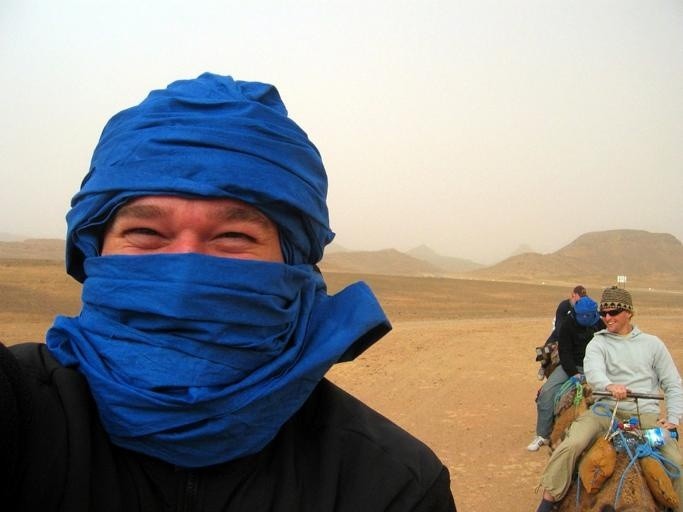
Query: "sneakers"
528 435 549 452
535 499 554 512
538 367 546 380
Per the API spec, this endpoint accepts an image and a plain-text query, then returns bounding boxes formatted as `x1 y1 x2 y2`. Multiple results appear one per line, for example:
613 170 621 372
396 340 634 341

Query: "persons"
0 71 454 511
534 284 682 511
526 297 607 457
537 284 590 384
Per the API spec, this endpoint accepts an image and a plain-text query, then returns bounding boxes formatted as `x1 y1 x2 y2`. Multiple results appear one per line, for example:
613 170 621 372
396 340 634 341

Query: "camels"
549 375 682 512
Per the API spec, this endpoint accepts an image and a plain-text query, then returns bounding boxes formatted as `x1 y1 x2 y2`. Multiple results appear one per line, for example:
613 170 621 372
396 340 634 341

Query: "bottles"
613 426 677 453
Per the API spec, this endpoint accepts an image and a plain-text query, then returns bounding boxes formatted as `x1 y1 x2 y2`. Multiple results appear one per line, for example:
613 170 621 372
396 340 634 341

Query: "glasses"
599 308 624 317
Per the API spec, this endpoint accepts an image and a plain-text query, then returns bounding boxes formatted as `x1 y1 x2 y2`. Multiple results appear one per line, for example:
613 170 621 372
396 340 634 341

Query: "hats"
573 286 588 297
600 286 633 313
